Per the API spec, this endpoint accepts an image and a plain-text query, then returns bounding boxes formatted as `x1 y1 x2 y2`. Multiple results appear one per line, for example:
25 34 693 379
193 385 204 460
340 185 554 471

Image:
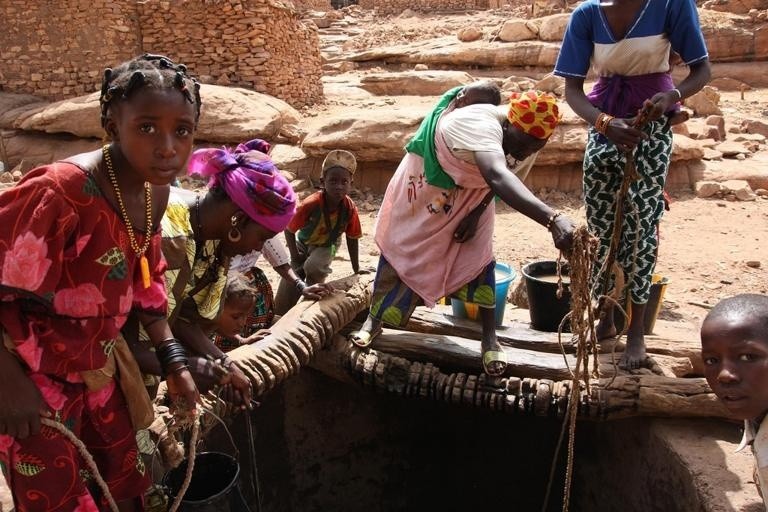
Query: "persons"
0 53 202 511
455 80 502 109
209 236 335 353
199 268 272 346
700 291 767 511
351 89 585 377
272 149 377 326
120 139 299 411
551 0 711 371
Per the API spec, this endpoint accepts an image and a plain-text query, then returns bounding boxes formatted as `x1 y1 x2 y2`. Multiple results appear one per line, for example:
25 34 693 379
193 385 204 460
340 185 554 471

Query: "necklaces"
194 189 202 261
99 143 155 260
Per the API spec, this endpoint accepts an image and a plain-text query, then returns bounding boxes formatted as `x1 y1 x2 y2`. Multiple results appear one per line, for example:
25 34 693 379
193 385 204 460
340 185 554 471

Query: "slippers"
481 341 509 379
351 325 383 347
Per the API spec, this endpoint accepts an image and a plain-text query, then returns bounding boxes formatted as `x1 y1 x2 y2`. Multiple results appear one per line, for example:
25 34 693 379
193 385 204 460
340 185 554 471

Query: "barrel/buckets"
613 274 671 334
451 260 516 325
521 259 574 332
149 406 240 512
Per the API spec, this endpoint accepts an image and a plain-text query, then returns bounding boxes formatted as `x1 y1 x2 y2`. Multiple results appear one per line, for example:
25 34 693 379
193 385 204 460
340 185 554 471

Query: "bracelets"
294 277 306 291
219 354 229 363
671 88 681 101
546 211 562 232
153 337 192 374
594 112 614 133
481 201 488 208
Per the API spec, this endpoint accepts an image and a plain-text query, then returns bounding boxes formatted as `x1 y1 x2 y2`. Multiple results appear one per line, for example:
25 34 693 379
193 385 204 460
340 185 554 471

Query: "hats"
320 149 358 177
